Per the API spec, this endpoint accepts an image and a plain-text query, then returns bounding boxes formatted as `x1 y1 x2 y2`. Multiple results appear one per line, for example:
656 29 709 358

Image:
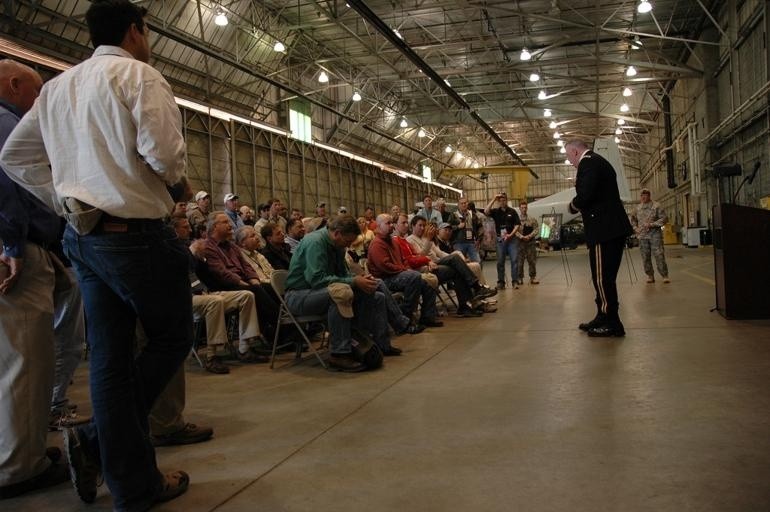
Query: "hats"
223 193 238 205
327 282 356 319
195 190 210 201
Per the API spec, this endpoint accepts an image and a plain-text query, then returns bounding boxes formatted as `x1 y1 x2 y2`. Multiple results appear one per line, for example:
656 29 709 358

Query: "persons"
175 190 498 372
630 188 671 284
134 172 214 447
563 137 633 338
484 192 521 289
51 243 91 433
0 56 71 500
0 0 191 512
515 202 539 285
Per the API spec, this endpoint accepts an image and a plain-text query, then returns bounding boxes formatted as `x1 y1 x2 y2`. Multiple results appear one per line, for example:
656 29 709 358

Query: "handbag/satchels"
349 326 384 370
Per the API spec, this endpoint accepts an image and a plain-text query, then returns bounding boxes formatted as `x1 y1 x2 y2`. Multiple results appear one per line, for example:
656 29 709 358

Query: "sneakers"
645 270 672 284
495 277 539 289
203 343 273 374
577 314 625 339
325 354 363 373
1 407 214 504
402 315 444 334
456 284 498 317
377 345 402 356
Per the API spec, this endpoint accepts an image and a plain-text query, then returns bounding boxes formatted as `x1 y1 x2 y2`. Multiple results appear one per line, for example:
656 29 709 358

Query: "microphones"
748 161 761 185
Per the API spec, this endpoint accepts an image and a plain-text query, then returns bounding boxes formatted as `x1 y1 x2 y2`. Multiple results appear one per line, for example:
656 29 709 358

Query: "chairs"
174 198 491 372
